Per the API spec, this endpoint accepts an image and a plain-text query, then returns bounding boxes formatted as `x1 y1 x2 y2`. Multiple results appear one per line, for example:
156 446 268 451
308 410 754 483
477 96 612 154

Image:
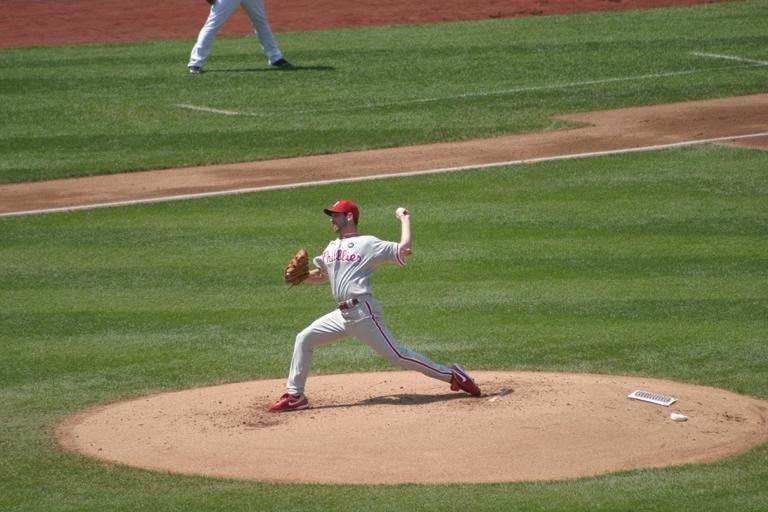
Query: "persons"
188 1 291 73
268 198 481 411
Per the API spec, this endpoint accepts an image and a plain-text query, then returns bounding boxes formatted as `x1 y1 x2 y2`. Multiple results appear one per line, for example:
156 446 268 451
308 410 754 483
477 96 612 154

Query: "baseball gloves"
284 250 310 286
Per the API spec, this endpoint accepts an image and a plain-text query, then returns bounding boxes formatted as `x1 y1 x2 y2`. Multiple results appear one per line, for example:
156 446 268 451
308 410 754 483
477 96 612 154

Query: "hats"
324 200 359 220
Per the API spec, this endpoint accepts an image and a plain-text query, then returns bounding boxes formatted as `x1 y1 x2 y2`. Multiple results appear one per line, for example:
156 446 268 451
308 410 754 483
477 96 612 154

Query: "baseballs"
396 207 406 215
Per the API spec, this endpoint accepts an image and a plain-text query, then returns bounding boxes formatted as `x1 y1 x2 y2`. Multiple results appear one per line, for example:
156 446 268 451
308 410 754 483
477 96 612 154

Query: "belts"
339 299 358 310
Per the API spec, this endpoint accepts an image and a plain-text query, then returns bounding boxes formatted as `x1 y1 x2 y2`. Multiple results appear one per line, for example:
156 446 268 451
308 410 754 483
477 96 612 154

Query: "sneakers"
271 59 293 71
267 393 310 412
191 66 200 75
451 362 480 397
